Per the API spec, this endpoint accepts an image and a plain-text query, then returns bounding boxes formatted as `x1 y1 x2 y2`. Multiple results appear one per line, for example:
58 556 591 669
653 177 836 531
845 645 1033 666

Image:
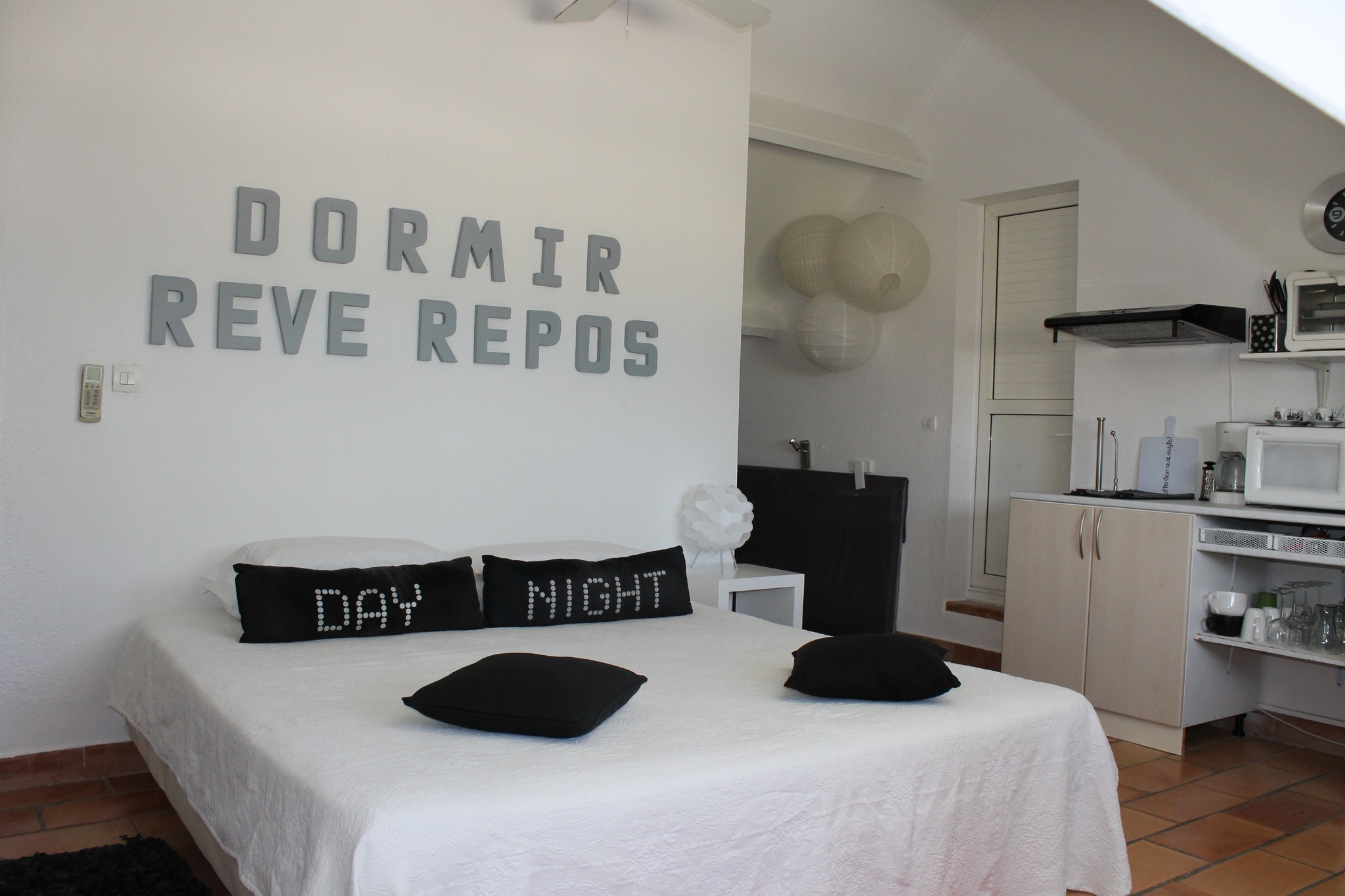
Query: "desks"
687 562 805 630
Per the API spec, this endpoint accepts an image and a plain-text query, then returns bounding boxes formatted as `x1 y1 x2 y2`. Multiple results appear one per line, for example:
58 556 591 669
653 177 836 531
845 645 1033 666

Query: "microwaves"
1244 425 1345 513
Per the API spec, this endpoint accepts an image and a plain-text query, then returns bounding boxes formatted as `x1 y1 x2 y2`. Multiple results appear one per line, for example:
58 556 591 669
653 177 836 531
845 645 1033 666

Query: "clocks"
1302 172 1345 254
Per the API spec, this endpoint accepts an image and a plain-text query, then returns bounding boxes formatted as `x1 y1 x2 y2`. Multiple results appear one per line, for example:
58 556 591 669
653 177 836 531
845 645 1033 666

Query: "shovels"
1269 270 1281 312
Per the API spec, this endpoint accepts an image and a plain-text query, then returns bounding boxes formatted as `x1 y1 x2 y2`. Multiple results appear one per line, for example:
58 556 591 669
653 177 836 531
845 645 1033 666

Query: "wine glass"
1266 580 1332 651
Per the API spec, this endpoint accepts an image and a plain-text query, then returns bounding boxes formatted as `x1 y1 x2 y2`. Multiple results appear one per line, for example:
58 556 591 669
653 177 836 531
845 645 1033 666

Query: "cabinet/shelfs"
1193 511 1345 671
1000 497 1261 757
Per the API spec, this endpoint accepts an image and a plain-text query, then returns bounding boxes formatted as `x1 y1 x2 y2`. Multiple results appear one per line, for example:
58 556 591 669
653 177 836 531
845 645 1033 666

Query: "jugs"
1212 452 1245 493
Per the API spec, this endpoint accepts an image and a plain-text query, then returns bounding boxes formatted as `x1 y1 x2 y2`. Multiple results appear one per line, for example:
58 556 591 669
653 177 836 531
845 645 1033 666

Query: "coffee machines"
1208 420 1270 505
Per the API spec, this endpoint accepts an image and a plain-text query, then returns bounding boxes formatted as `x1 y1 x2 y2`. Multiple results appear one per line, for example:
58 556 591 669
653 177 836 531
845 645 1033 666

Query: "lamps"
779 206 931 372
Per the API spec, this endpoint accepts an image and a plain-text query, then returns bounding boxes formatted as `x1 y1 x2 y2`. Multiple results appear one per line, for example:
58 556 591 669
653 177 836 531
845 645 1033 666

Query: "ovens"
1284 270 1345 351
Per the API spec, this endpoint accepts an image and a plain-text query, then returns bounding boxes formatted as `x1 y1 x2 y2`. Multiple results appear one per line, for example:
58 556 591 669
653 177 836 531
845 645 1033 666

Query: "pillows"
200 535 454 621
481 545 693 625
228 555 482 644
400 651 648 740
783 628 962 702
455 542 644 576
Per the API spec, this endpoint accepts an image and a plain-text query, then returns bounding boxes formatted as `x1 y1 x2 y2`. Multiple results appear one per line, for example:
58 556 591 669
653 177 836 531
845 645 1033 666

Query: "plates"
1265 420 1300 426
1306 420 1343 427
1293 421 1310 427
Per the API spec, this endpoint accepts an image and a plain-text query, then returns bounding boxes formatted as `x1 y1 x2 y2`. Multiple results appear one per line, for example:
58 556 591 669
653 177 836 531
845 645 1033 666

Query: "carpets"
0 835 215 896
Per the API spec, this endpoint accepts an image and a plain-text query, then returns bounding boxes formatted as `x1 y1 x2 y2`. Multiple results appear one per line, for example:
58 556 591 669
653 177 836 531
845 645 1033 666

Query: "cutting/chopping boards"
1137 416 1199 495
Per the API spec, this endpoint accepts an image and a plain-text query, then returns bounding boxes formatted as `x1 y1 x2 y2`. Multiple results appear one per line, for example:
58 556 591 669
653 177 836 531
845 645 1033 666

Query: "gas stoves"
1063 488 1196 499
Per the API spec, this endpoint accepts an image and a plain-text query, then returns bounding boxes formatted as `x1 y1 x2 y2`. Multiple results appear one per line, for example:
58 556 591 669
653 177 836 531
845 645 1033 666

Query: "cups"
1262 606 1281 624
1309 405 1345 423
1307 600 1345 661
1240 608 1266 643
1290 409 1303 421
1296 605 1312 617
1274 407 1291 420
1205 614 1244 637
1278 606 1293 619
1207 591 1248 616
1257 591 1278 609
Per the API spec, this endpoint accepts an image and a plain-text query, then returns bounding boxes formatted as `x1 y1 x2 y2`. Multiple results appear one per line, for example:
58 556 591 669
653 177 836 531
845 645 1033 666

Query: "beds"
110 610 1137 896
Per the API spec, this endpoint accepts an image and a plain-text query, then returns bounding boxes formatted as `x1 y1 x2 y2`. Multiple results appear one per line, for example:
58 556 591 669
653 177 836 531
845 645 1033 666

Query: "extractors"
1044 303 1246 348
